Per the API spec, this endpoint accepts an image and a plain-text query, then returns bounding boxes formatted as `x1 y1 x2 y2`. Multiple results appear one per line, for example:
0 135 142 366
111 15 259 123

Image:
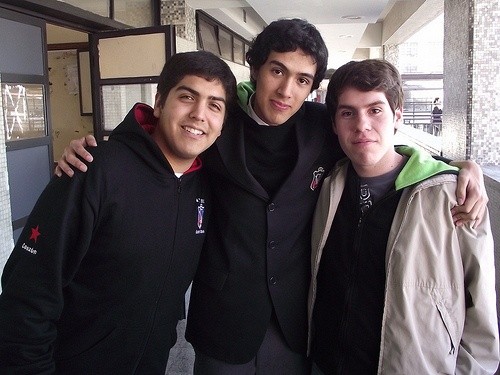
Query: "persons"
0 50 240 375
432 97 443 136
55 18 490 375
303 59 500 375
